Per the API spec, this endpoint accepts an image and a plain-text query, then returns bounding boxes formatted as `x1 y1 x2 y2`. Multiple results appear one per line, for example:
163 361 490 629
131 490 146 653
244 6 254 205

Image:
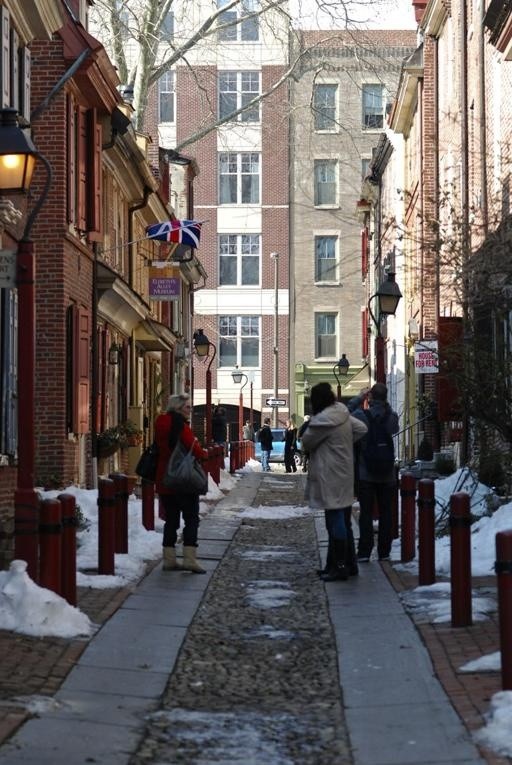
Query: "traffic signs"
265 398 286 406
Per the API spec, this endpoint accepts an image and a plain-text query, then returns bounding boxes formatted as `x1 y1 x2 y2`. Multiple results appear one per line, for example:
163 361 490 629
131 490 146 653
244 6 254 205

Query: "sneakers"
357 551 370 562
265 469 274 472
378 554 392 561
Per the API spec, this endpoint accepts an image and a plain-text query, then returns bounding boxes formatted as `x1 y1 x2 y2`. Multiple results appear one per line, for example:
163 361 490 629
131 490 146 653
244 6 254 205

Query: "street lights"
192 328 217 448
0 105 53 591
269 251 280 430
230 365 249 442
332 352 350 401
365 271 402 402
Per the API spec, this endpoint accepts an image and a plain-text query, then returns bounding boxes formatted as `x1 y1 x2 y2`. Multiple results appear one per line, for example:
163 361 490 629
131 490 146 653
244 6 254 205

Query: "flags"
146 220 202 248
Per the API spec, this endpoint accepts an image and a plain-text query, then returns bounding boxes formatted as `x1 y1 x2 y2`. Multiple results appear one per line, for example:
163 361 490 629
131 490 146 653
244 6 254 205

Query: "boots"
182 544 206 572
163 545 184 570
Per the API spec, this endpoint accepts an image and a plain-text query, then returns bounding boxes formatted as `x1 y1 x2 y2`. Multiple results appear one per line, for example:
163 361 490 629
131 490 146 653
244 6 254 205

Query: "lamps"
108 338 121 365
102 101 141 151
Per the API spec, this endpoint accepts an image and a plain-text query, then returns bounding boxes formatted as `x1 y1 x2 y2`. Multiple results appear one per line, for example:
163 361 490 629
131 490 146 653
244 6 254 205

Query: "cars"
254 428 306 467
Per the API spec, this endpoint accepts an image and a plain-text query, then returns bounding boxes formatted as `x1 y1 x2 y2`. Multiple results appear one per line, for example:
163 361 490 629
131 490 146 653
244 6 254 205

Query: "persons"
155 393 209 574
260 418 273 472
282 384 397 582
212 408 227 443
243 420 254 440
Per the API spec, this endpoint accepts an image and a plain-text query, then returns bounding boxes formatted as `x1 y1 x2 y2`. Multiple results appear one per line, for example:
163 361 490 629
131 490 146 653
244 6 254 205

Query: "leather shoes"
319 562 358 581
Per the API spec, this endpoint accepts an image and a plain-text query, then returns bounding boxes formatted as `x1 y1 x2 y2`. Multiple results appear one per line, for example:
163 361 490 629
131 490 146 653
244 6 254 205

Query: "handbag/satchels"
136 442 158 482
158 435 188 502
174 436 209 494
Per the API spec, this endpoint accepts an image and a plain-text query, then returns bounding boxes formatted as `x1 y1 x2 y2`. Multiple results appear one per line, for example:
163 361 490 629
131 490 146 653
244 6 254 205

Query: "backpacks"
256 426 266 442
363 407 395 472
